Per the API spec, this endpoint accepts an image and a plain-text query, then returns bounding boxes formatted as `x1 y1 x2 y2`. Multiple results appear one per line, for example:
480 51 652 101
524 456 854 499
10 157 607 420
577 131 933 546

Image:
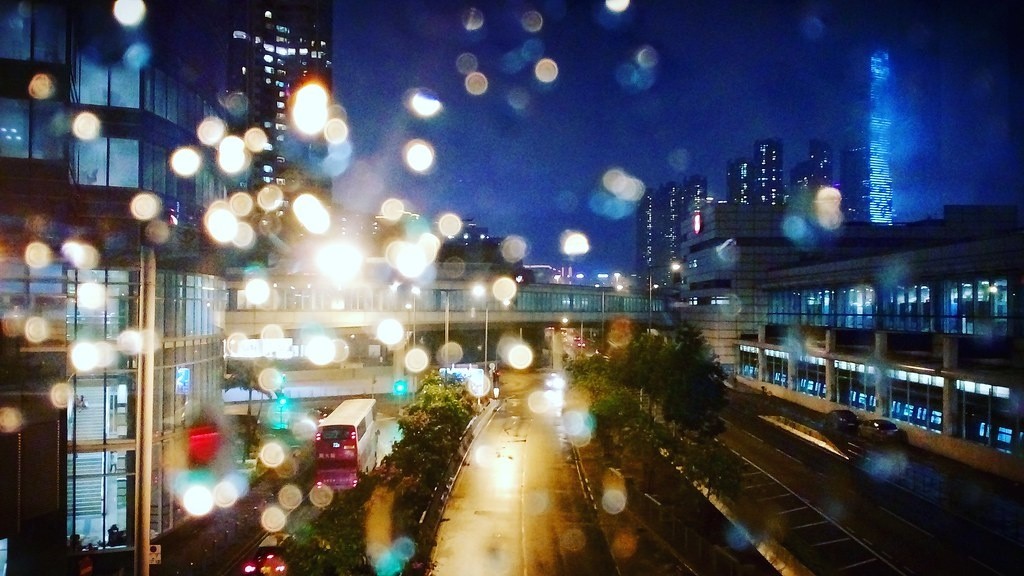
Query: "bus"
314 399 381 495
